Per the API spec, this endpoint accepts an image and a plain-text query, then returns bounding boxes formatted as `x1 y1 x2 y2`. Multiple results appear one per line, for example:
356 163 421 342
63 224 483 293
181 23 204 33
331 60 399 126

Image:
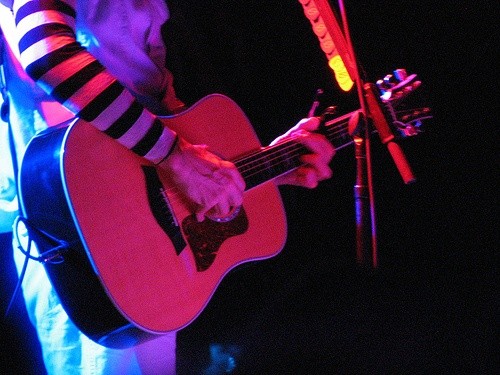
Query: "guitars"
16 66 433 350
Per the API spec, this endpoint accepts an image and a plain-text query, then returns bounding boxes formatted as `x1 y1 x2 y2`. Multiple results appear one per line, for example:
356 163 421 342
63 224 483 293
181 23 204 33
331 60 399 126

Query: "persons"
0 0 336 375
